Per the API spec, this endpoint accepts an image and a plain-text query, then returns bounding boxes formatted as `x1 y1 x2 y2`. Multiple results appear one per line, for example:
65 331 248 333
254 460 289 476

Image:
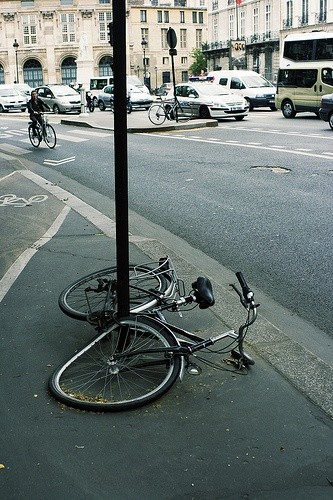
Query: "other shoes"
33 130 37 135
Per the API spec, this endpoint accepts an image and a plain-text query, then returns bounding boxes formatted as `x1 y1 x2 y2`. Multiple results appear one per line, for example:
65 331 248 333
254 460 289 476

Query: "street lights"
141 37 148 85
13 39 19 84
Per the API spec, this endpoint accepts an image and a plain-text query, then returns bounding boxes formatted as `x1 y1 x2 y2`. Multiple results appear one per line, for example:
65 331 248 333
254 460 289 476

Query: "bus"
275 29 333 119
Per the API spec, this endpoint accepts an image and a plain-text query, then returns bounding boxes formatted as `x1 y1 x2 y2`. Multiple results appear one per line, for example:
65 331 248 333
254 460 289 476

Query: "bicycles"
147 94 194 126
28 109 57 149
47 254 261 414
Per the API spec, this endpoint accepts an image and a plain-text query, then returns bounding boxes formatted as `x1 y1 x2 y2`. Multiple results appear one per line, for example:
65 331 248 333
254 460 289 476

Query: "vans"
90 75 154 115
207 70 278 112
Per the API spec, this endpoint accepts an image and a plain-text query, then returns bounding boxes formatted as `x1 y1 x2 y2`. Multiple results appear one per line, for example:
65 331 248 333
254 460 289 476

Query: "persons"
28 90 52 143
69 82 78 91
77 83 83 93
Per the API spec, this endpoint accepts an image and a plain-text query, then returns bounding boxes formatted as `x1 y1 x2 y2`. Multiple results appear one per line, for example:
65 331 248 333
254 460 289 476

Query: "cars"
159 82 177 95
318 92 333 131
34 83 85 115
164 76 250 121
0 84 33 114
